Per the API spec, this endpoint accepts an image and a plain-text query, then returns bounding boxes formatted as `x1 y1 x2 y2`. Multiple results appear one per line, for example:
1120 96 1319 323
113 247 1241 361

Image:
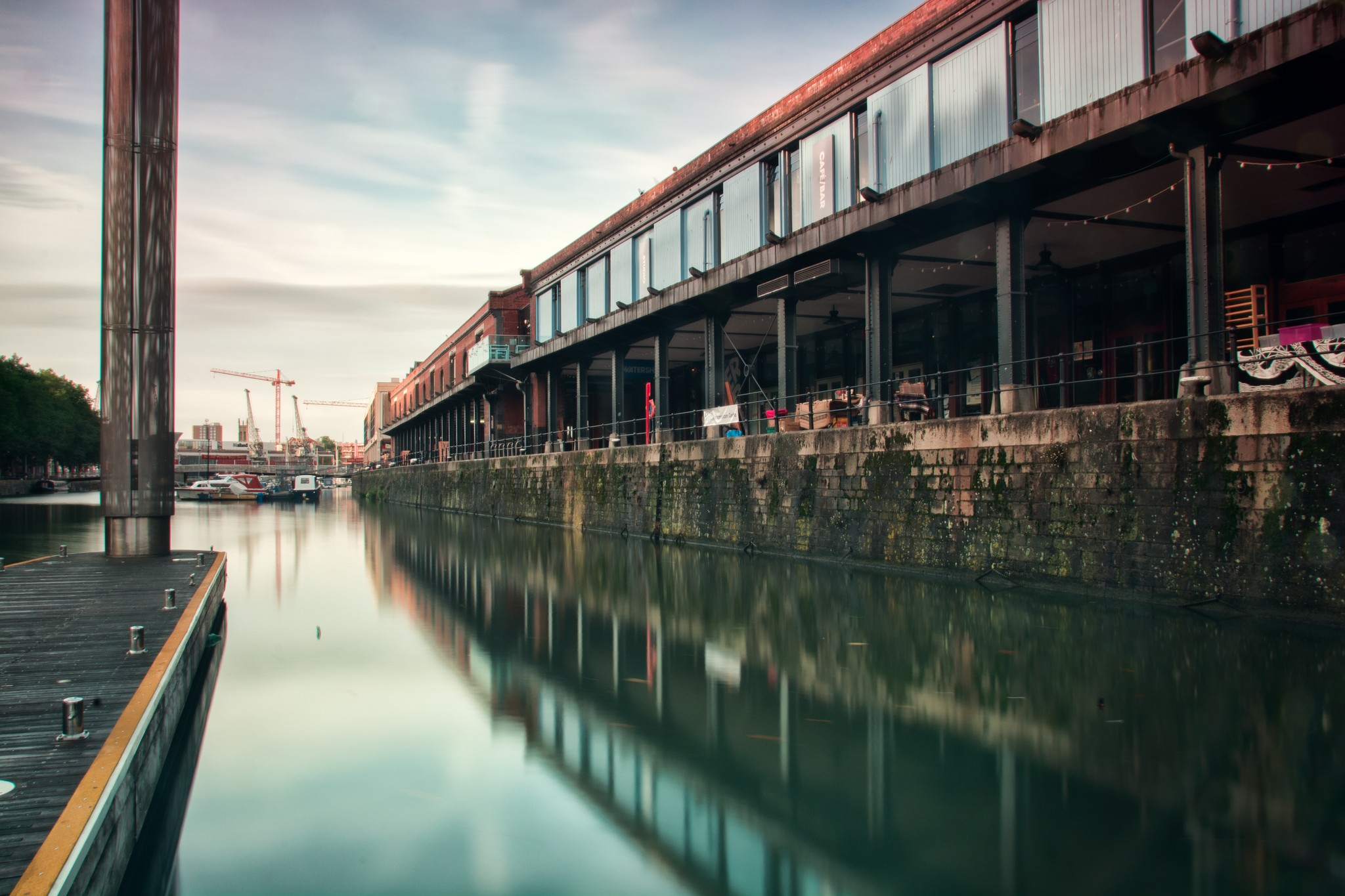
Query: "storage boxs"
1258 324 1345 348
767 418 801 434
832 418 848 428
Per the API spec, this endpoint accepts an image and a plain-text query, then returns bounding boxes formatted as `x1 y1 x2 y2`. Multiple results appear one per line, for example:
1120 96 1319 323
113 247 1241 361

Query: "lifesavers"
645 399 656 418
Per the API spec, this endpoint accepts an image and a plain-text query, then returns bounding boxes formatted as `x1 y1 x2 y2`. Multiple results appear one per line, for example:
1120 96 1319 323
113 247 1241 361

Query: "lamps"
616 301 631 310
461 374 469 381
859 187 886 203
1190 31 1229 63
585 318 599 324
379 404 423 431
424 399 430 402
510 351 519 357
1009 118 1042 143
765 232 785 245
555 330 567 337
446 384 452 390
435 392 441 396
533 340 545 347
647 287 663 296
688 267 707 279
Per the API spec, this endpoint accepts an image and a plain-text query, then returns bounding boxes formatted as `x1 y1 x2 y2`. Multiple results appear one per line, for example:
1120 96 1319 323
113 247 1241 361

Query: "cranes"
303 397 369 409
208 365 295 452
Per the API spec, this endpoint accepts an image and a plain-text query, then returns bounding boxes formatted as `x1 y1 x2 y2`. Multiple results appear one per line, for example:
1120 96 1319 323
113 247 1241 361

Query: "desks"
795 399 847 430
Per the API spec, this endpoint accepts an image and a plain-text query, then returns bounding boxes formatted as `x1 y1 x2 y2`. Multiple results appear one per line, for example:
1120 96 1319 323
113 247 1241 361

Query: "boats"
30 480 71 494
175 472 353 503
